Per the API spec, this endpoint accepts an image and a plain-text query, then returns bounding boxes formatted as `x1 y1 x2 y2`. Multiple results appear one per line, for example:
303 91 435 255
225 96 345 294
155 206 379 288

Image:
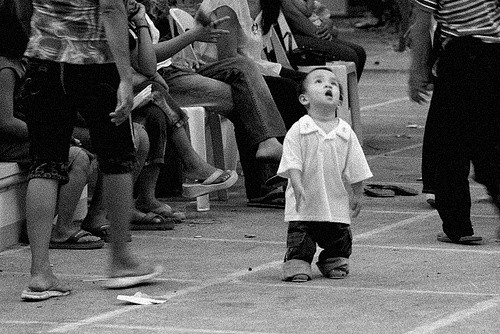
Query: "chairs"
169 7 200 66
270 11 364 148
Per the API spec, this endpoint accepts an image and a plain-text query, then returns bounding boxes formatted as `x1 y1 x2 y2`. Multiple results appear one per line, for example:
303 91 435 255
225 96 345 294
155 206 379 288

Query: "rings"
212 21 216 28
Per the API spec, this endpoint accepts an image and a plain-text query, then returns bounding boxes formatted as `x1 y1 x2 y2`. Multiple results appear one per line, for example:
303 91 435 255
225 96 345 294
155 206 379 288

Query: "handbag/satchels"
287 46 326 66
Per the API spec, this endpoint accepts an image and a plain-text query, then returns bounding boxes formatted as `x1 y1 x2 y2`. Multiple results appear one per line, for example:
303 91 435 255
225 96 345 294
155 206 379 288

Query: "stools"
0 162 88 252
180 107 228 211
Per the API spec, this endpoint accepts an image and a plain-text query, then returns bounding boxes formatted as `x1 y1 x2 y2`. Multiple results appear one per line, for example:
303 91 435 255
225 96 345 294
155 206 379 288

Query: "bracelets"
135 25 149 29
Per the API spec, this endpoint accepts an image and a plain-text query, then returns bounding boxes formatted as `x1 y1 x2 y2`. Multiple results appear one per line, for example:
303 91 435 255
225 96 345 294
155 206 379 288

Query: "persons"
21 0 164 299
0 0 366 249
276 66 374 282
408 0 500 245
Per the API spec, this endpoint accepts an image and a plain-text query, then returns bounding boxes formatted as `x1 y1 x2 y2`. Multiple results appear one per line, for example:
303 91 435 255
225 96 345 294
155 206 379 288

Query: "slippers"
49 229 104 250
129 212 175 231
437 232 482 242
80 223 132 243
246 191 285 209
106 265 164 289
21 278 73 299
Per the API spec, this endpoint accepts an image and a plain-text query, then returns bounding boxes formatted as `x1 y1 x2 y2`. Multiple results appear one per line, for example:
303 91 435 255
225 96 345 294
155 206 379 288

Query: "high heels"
182 168 239 198
151 204 185 221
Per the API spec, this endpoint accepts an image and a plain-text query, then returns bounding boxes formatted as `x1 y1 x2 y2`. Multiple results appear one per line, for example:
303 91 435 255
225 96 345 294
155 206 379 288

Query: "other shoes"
292 275 308 282
328 269 347 279
